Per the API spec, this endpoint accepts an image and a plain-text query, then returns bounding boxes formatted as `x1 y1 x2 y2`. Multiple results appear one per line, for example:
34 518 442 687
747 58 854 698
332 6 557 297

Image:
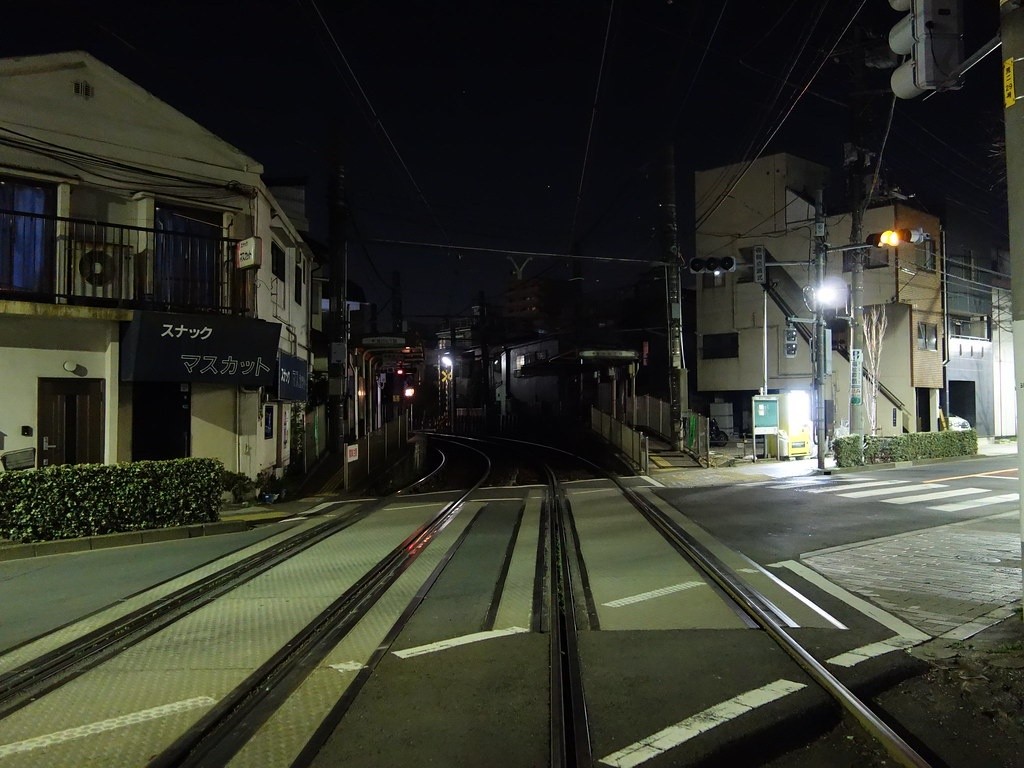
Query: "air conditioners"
69 240 134 300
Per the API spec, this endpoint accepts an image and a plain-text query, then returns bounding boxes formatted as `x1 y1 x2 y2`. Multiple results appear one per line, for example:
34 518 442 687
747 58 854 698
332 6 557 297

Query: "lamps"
62 360 77 372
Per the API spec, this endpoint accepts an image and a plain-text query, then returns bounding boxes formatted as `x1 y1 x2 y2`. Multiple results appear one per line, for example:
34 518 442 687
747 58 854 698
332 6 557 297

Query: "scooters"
708 418 729 447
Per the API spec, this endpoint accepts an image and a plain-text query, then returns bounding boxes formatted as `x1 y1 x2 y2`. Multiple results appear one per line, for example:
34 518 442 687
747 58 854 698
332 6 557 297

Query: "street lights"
440 355 456 435
813 272 843 469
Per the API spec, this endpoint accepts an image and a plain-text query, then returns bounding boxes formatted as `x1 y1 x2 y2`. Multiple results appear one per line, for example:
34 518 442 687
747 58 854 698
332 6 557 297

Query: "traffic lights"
873 228 923 248
783 327 799 359
887 0 966 101
688 255 737 274
